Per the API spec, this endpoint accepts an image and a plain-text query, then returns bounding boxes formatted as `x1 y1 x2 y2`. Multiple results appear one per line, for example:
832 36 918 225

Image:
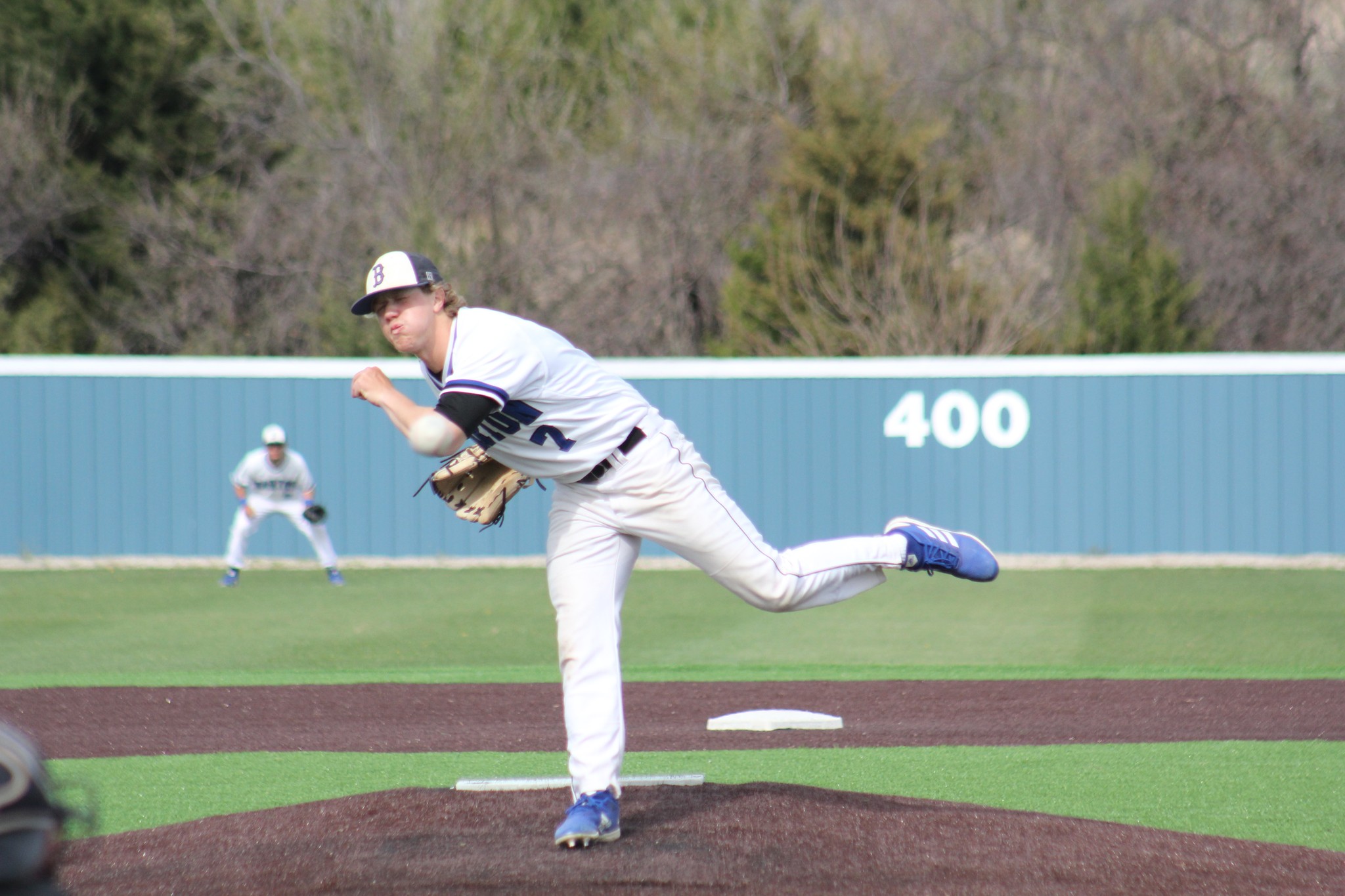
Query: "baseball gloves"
426 448 537 527
303 505 325 524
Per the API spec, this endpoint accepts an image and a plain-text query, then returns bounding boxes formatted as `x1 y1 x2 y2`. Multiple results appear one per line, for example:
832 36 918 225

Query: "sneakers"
556 793 623 849
886 514 1001 586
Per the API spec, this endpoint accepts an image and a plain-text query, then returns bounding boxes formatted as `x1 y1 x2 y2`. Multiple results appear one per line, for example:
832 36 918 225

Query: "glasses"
266 442 282 448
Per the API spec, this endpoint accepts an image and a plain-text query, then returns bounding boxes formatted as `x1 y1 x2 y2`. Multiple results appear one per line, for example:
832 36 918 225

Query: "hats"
350 252 442 317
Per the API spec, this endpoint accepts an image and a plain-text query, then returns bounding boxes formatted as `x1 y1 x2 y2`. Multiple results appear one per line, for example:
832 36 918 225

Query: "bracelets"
304 500 314 507
238 498 246 507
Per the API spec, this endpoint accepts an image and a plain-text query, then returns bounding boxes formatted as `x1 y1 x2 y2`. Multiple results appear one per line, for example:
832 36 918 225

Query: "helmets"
263 423 285 442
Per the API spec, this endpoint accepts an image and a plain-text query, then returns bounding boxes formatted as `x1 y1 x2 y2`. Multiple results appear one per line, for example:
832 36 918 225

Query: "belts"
577 426 646 484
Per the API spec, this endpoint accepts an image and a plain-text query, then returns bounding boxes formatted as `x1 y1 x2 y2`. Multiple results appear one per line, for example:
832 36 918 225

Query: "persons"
222 423 344 588
349 250 1000 847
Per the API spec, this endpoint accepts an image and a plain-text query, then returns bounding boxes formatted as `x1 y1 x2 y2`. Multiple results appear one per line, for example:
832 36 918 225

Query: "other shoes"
326 567 342 586
226 569 240 586
